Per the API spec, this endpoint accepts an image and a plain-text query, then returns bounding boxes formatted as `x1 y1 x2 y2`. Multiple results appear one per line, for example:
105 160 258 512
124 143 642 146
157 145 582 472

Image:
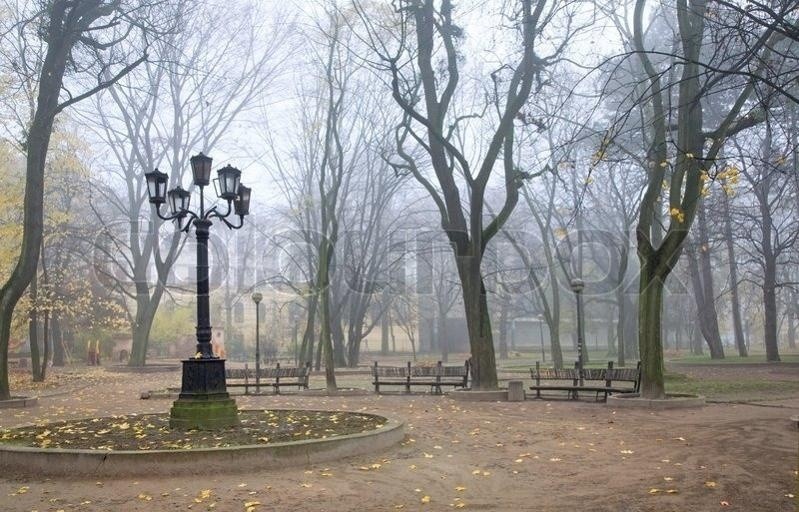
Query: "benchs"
225 362 312 394
371 361 469 392
529 361 643 404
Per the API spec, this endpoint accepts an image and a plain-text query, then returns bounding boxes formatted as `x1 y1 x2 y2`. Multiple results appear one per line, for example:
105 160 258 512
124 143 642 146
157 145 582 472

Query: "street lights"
144 153 251 430
570 278 585 388
253 292 263 393
